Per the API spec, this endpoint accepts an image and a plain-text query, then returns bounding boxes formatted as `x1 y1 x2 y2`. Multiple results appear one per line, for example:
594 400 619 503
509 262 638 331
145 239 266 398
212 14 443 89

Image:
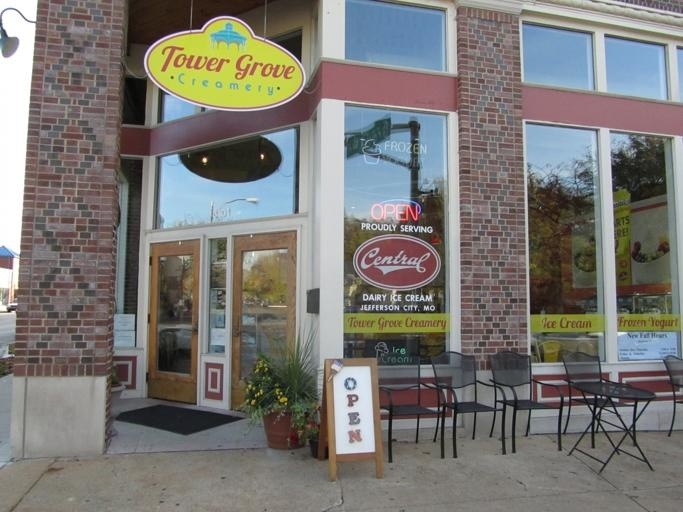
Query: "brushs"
327 359 343 382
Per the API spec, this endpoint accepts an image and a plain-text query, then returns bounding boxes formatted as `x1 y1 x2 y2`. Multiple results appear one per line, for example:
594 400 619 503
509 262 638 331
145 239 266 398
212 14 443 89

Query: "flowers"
235 320 321 449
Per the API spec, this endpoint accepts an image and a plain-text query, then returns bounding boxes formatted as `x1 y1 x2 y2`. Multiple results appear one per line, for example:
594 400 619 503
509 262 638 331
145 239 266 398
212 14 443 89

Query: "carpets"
112 403 246 436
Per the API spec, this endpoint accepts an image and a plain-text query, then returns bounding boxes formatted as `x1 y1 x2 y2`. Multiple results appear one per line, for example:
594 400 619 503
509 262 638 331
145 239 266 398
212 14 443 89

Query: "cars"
157 309 270 381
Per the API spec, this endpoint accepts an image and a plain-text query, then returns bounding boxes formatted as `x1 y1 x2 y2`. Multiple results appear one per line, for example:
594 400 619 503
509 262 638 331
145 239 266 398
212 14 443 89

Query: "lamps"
0 8 36 60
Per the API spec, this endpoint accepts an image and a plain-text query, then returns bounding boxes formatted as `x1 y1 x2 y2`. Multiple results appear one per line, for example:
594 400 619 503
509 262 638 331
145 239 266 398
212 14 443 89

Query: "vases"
259 409 310 449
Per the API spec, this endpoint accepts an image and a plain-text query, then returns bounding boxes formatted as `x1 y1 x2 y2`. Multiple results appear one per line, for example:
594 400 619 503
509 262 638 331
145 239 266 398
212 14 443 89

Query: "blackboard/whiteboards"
364 339 420 366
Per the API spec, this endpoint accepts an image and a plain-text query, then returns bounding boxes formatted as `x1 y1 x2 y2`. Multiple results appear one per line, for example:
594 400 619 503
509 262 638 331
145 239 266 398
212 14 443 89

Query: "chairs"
375 349 638 462
663 355 682 436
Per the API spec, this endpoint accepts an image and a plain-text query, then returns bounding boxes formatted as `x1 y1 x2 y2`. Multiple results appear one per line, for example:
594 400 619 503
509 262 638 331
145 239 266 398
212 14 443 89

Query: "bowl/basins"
631 251 669 285
572 253 596 286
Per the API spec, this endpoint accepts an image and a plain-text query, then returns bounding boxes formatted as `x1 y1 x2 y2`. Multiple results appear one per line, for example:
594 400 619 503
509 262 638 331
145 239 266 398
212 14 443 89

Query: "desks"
567 380 656 474
